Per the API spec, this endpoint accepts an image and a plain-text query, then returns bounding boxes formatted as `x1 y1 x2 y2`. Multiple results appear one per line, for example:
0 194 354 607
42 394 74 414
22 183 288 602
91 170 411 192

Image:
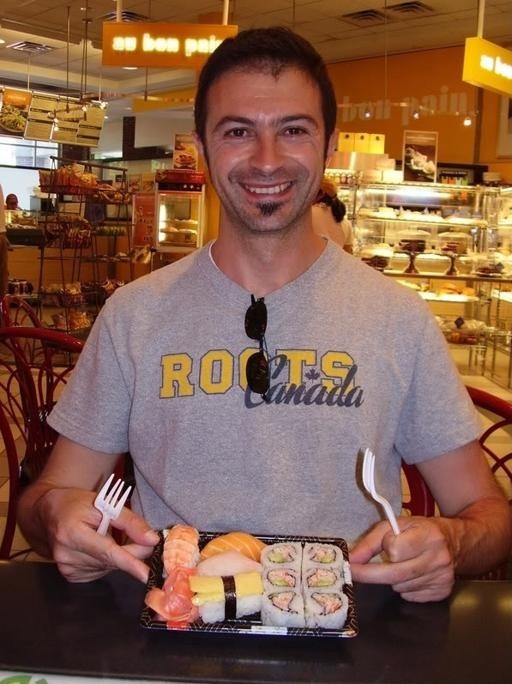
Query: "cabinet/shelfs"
0 155 512 422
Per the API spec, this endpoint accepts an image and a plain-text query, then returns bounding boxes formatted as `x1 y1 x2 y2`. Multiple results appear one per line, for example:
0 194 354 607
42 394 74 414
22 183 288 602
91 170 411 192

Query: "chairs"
0 292 512 582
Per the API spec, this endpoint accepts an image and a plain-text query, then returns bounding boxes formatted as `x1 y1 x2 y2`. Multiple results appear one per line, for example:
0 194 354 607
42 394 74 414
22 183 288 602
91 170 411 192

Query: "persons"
1 184 18 307
4 191 25 212
19 19 511 606
308 171 354 256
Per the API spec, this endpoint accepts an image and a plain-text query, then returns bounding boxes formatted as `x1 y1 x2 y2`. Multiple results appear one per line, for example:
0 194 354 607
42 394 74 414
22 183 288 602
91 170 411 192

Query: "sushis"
260 539 349 630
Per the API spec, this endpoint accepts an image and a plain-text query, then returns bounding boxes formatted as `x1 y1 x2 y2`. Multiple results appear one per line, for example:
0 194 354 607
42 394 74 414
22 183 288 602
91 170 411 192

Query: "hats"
321 176 337 197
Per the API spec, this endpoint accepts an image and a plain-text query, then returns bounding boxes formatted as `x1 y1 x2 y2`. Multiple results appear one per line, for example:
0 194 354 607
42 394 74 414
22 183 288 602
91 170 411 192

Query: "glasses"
245 295 270 404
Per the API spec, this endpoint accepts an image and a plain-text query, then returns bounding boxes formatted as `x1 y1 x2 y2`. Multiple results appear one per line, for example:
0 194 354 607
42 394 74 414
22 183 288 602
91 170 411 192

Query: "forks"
358 447 402 536
94 474 131 536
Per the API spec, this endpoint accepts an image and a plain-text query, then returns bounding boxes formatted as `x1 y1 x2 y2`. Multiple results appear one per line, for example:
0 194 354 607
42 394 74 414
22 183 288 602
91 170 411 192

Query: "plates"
139 530 359 640
0 106 28 134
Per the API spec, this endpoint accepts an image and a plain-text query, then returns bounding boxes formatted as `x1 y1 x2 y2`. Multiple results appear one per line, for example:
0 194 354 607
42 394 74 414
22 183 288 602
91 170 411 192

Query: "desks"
0 557 512 684
